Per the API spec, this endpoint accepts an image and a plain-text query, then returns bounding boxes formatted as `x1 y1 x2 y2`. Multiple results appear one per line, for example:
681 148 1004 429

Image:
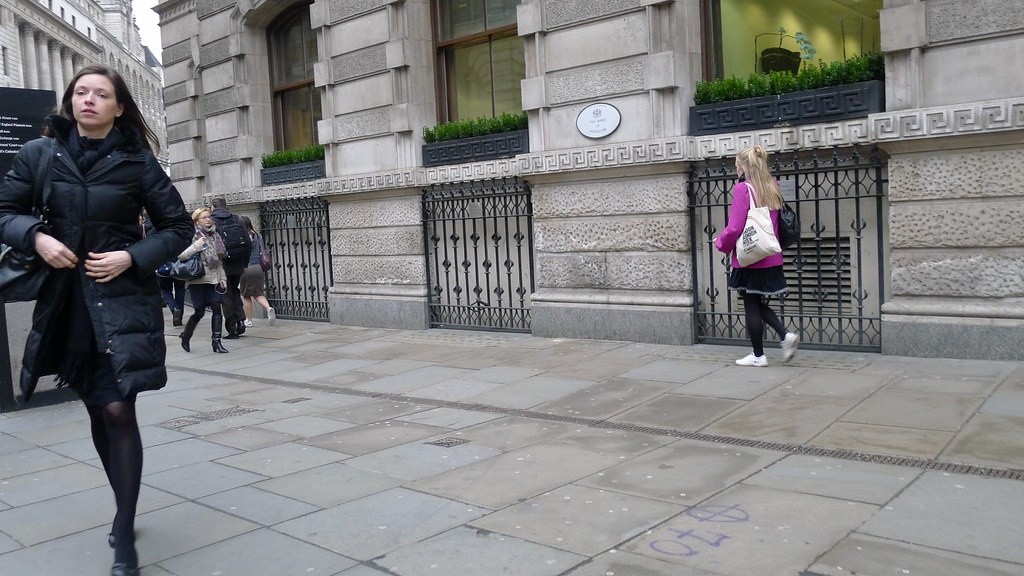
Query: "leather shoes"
237 321 246 336
224 332 238 339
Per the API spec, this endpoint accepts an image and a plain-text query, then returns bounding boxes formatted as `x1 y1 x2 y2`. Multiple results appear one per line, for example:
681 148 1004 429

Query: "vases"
760 47 801 75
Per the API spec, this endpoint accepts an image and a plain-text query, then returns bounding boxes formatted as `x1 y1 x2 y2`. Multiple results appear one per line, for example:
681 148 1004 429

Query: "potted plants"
258 143 327 186
420 112 533 168
686 52 883 137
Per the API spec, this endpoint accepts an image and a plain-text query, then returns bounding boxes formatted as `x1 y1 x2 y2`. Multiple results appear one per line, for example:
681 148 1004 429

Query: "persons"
715 146 798 367
209 198 252 339
0 65 195 576
238 216 276 328
177 207 228 353
138 209 186 326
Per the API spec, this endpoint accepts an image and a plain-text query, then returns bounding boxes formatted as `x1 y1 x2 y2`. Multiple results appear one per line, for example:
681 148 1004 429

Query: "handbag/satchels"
777 194 799 249
0 137 53 302
734 183 783 269
258 235 272 271
169 238 205 279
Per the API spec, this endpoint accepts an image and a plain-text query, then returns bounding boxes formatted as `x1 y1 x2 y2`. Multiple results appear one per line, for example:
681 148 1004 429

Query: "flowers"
776 25 817 59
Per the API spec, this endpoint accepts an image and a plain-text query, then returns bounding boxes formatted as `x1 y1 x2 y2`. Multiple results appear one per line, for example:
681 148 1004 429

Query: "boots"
179 319 199 352
212 314 228 353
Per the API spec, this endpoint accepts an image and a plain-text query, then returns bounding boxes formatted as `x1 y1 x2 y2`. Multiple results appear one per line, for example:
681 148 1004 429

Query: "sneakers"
736 354 767 368
781 333 799 363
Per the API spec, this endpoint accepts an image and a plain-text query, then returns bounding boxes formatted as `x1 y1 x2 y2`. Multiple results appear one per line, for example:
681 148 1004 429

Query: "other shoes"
173 306 182 327
267 306 276 326
111 552 139 576
109 532 117 548
243 319 251 327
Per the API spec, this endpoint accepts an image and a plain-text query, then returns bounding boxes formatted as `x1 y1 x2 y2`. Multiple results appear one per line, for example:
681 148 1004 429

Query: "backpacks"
214 214 252 261
